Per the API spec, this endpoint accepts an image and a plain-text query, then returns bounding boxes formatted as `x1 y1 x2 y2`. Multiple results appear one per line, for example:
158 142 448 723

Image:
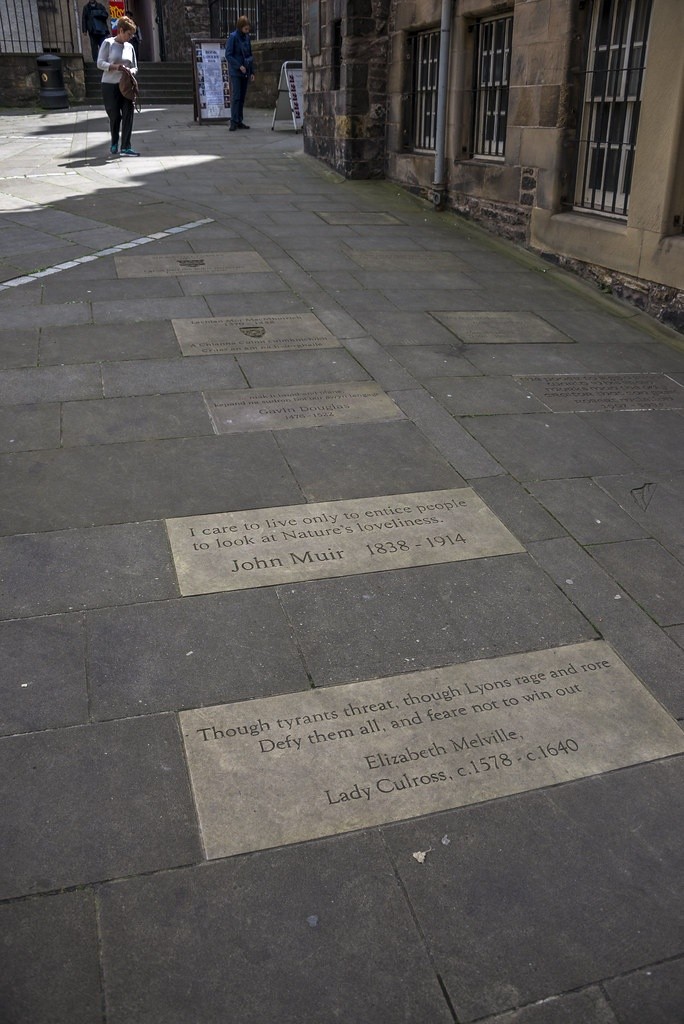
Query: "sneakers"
110 144 118 154
120 149 140 157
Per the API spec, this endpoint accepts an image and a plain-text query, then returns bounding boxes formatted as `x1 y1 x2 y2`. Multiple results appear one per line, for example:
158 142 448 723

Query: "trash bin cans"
37 55 68 109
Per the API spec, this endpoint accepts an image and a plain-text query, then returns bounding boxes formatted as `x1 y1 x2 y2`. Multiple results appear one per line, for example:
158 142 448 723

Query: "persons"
97 16 140 157
224 16 255 131
81 0 143 64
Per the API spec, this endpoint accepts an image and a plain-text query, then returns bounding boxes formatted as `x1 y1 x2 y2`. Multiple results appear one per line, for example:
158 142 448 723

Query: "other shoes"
228 120 238 131
94 61 97 64
238 123 250 129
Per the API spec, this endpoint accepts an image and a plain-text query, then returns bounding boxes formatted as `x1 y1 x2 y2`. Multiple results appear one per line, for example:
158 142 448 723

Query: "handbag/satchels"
120 66 141 114
